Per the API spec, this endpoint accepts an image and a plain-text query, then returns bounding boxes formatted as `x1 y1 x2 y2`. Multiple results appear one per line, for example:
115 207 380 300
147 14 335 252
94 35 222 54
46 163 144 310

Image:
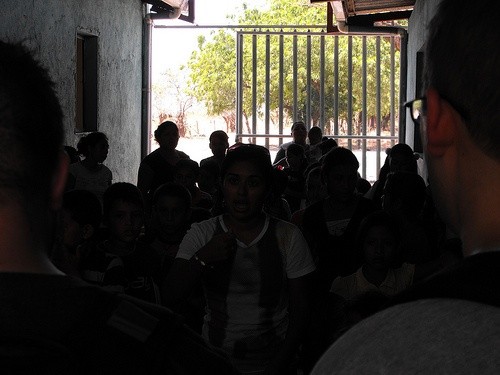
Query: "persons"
46 120 463 375
0 39 241 375
311 0 500 375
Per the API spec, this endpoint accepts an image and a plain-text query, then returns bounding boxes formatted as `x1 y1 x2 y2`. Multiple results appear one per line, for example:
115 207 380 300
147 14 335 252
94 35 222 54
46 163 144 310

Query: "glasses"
406 97 425 125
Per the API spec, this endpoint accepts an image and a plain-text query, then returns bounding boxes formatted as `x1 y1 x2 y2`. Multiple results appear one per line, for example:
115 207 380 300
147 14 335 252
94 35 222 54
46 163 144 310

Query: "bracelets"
191 254 215 272
